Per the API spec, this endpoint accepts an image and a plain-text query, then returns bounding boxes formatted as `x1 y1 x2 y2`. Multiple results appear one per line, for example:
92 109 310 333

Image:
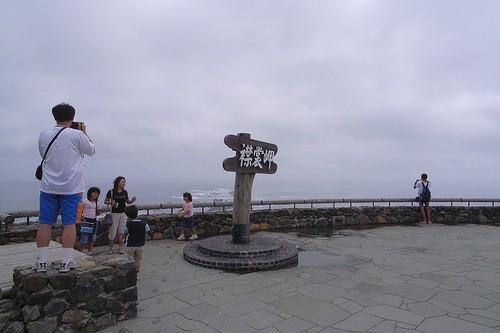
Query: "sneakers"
59 256 78 272
32 257 48 272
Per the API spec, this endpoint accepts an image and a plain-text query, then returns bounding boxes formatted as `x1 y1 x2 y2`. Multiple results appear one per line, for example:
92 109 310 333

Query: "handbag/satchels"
35 164 42 181
104 213 114 225
79 221 94 234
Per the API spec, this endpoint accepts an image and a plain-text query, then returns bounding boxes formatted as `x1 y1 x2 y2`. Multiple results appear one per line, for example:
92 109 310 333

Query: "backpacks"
421 181 431 201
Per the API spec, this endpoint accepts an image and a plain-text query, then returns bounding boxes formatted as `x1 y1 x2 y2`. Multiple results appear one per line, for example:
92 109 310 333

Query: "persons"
104 176 137 255
74 192 83 252
177 192 198 240
119 205 150 280
414 174 432 224
79 186 102 256
32 102 96 272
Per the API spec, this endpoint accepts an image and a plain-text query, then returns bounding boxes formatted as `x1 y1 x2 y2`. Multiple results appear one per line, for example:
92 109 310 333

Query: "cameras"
70 122 83 130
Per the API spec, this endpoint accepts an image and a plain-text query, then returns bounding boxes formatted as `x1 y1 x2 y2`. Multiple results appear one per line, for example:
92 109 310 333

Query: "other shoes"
107 247 114 254
189 235 198 240
177 234 186 240
118 248 124 254
